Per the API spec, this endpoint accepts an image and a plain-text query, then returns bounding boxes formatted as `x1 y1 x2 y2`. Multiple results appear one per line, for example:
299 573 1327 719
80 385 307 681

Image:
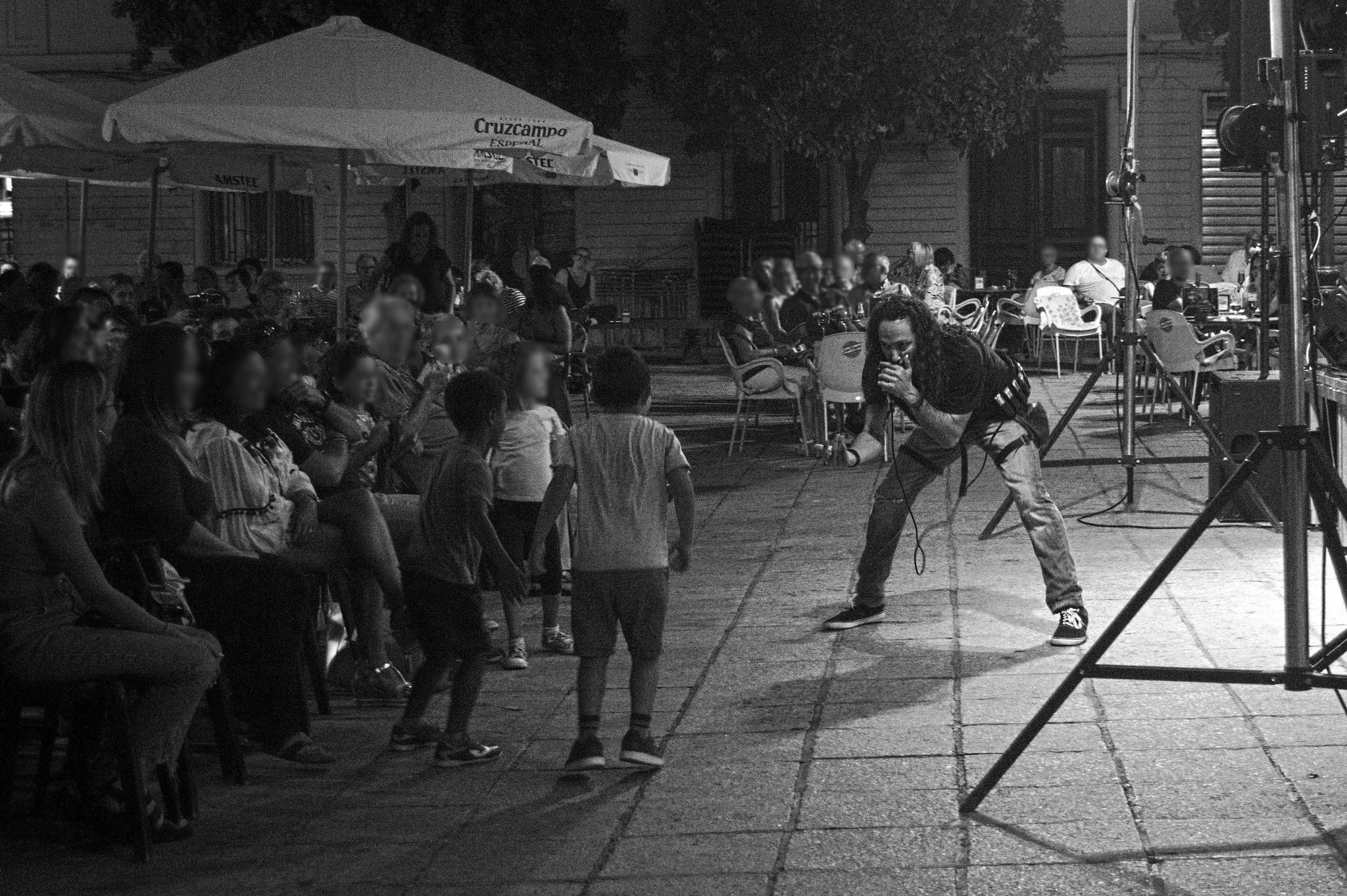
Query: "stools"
0 679 196 864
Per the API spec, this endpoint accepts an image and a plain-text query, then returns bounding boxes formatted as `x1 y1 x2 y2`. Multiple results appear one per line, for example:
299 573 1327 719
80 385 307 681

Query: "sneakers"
564 737 606 769
823 598 887 629
1050 606 1088 647
389 720 445 750
541 626 574 655
432 733 502 767
621 729 665 766
505 638 528 670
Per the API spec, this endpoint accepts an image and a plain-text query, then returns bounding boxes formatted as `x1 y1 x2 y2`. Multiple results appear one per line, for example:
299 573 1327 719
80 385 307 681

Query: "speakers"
1239 1 1347 174
1210 368 1288 523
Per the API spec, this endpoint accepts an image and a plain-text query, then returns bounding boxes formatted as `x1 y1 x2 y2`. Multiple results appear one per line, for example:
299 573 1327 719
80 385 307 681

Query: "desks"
955 279 1033 360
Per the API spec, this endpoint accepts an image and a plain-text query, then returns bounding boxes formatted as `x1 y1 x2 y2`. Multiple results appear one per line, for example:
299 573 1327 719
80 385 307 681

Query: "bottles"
1005 269 1014 290
857 301 865 320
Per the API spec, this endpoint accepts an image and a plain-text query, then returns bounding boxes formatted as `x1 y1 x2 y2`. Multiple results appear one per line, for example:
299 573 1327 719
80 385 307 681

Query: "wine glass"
1243 293 1249 320
1194 271 1201 288
1238 270 1245 294
1231 304 1241 316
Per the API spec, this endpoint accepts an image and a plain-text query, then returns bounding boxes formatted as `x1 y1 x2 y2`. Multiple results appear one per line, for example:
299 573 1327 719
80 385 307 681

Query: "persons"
1061 233 1128 354
0 212 695 837
823 294 1089 649
1140 228 1279 418
723 240 980 459
1016 241 1068 318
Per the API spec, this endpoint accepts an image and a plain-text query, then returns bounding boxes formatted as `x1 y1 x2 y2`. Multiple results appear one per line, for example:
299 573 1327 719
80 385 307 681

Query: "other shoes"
486 644 505 662
96 780 180 843
354 660 412 706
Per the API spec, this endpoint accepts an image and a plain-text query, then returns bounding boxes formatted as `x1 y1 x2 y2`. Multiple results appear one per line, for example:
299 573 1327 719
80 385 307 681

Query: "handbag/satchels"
96 538 196 630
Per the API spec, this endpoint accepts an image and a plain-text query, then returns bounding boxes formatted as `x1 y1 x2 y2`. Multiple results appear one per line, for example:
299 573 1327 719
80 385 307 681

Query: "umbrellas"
99 11 596 347
0 63 163 187
348 134 672 296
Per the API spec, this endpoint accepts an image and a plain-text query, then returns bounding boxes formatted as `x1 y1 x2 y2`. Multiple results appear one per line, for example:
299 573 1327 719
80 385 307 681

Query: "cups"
621 312 631 323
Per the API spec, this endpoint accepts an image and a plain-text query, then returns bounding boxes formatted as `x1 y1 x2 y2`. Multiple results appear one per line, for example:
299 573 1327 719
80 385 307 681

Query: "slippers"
255 726 336 764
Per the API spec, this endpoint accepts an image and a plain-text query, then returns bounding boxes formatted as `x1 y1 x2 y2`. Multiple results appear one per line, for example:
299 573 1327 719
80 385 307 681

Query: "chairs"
559 320 593 421
711 284 1240 468
